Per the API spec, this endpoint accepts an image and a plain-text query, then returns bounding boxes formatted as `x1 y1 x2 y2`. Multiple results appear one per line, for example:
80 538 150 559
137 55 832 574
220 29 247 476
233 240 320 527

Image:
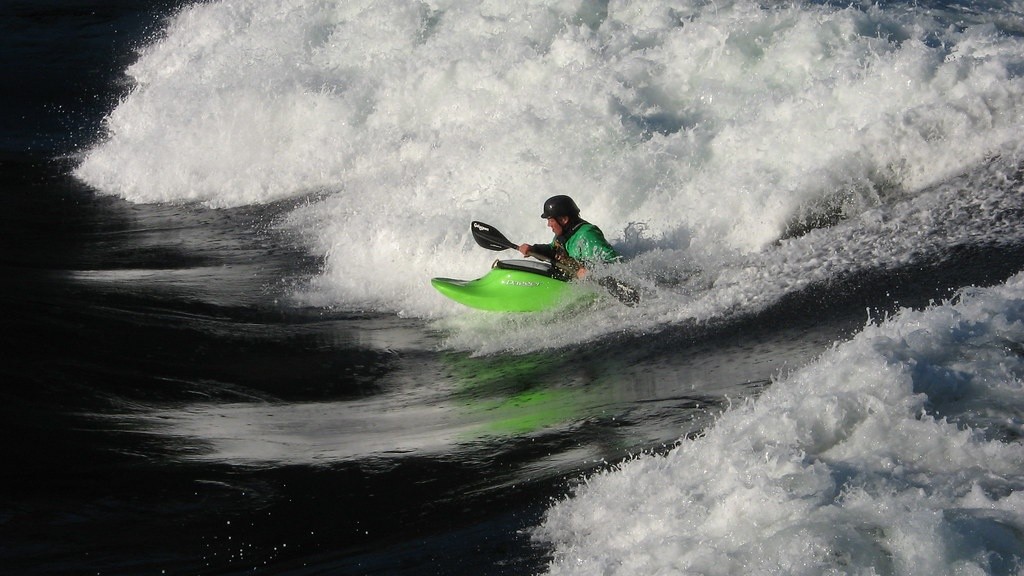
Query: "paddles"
471 219 639 308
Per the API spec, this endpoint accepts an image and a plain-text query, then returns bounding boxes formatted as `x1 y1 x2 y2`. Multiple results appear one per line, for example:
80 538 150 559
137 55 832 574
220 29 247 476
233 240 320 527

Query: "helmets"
541 195 580 218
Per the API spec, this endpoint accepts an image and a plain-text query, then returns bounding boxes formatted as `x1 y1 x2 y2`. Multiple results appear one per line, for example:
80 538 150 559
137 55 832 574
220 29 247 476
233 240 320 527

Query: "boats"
431 260 605 325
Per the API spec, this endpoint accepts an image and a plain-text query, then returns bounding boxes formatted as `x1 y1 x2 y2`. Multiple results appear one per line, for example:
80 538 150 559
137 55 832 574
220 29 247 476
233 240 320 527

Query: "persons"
520 195 618 298
